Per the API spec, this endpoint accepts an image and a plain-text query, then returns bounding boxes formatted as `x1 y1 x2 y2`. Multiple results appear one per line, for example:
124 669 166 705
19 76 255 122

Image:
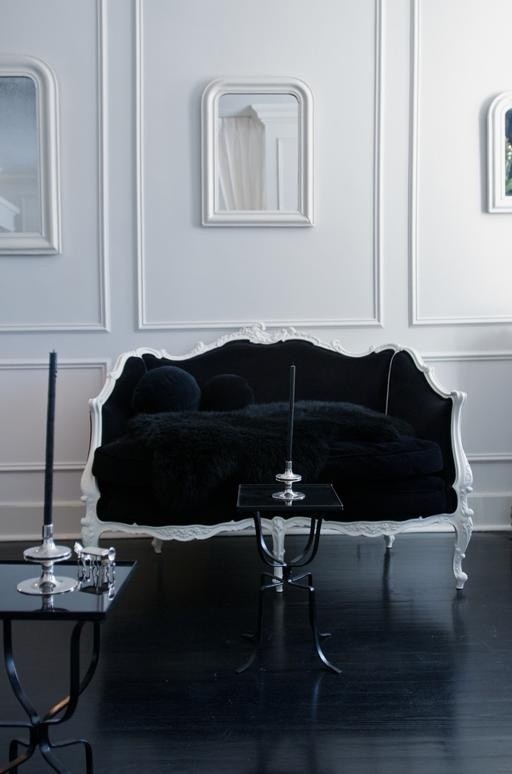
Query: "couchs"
79 320 475 593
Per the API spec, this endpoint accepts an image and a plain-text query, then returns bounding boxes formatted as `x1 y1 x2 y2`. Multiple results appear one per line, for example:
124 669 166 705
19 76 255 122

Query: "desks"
0 558 138 773
233 483 344 676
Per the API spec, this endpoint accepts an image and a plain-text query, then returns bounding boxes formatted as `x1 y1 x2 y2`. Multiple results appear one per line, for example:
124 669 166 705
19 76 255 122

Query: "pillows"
132 365 256 416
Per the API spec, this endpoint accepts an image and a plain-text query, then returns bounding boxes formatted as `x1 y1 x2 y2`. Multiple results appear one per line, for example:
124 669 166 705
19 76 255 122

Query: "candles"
286 364 298 462
43 349 57 525
